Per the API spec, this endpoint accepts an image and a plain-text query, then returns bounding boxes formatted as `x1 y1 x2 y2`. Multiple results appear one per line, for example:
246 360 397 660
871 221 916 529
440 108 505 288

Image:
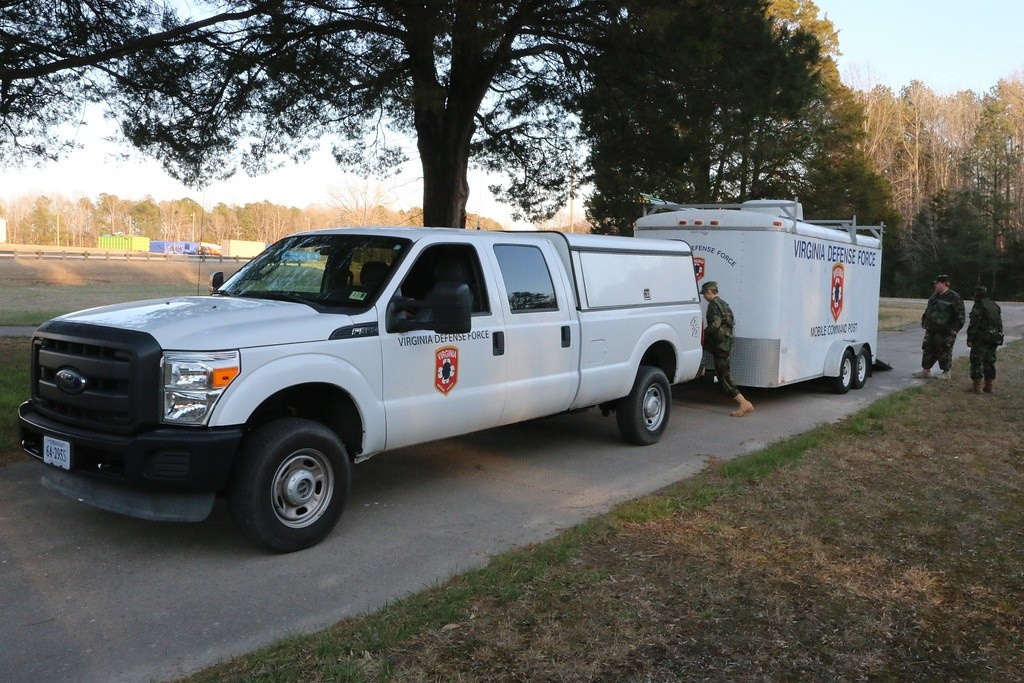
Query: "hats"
700 281 718 294
933 275 950 283
972 286 987 295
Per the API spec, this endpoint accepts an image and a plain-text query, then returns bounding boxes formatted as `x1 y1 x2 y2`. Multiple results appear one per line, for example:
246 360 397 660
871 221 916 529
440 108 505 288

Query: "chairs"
409 258 479 309
358 261 388 289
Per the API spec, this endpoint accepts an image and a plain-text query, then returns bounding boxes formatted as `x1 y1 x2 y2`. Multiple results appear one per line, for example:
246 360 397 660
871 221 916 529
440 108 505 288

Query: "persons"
911 275 966 381
701 281 754 418
967 286 1003 395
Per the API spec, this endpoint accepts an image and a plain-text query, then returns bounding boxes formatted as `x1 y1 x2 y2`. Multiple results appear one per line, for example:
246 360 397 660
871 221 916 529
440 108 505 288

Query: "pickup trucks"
17 227 703 554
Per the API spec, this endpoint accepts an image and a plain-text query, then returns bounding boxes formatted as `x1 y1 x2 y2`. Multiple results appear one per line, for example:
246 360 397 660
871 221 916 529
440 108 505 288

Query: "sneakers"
935 370 952 380
912 369 933 378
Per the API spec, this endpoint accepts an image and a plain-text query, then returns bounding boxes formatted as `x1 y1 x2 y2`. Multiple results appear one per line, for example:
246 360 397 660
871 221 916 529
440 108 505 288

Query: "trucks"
222 240 284 259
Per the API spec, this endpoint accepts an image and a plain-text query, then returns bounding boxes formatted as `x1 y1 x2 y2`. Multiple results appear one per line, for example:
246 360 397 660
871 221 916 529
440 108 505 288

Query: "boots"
968 379 994 393
730 394 754 417
